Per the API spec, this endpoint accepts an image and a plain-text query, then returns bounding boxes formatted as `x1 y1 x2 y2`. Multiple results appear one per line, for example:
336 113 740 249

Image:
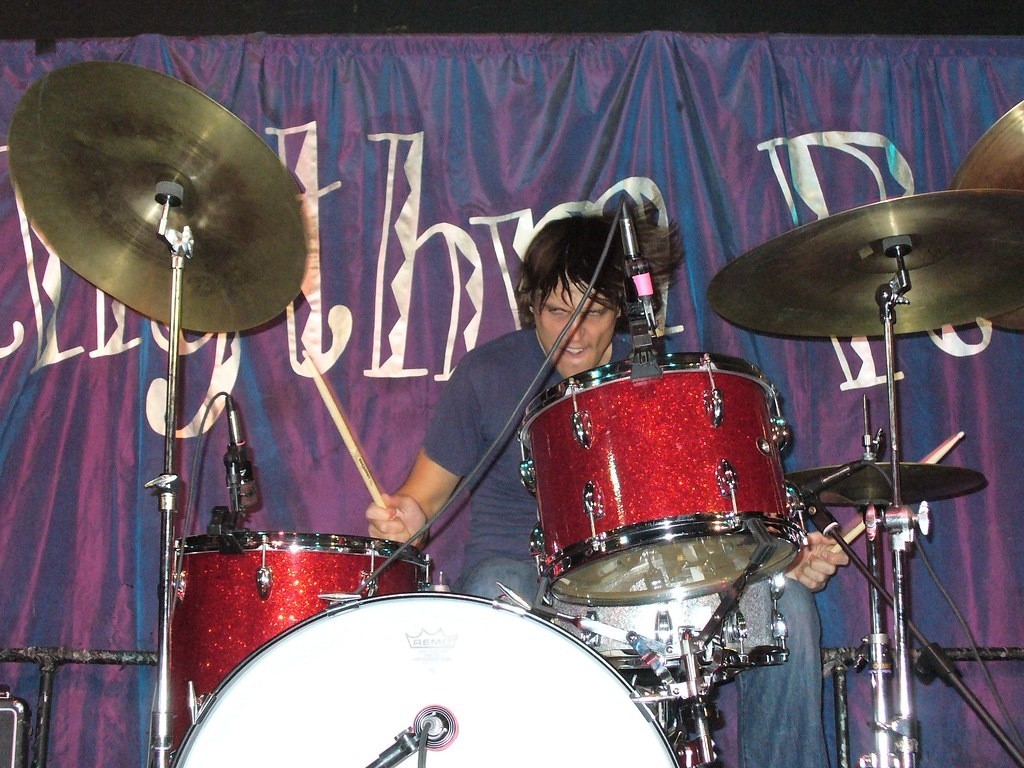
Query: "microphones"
223 396 259 508
415 704 459 749
799 460 867 500
619 200 662 317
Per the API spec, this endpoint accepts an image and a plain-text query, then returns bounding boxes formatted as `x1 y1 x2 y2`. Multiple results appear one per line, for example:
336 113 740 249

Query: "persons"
363 202 848 767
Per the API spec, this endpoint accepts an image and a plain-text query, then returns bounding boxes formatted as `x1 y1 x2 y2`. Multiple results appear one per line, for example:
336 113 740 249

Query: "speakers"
0 697 29 768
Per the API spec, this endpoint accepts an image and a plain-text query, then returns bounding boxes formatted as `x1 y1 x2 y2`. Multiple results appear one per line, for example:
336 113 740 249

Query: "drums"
168 529 432 767
170 584 683 768
518 350 809 609
542 574 791 671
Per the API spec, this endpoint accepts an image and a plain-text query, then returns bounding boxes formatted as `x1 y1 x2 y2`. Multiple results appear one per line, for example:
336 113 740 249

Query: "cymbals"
8 59 310 334
946 99 1024 332
785 460 987 509
706 187 1024 340
